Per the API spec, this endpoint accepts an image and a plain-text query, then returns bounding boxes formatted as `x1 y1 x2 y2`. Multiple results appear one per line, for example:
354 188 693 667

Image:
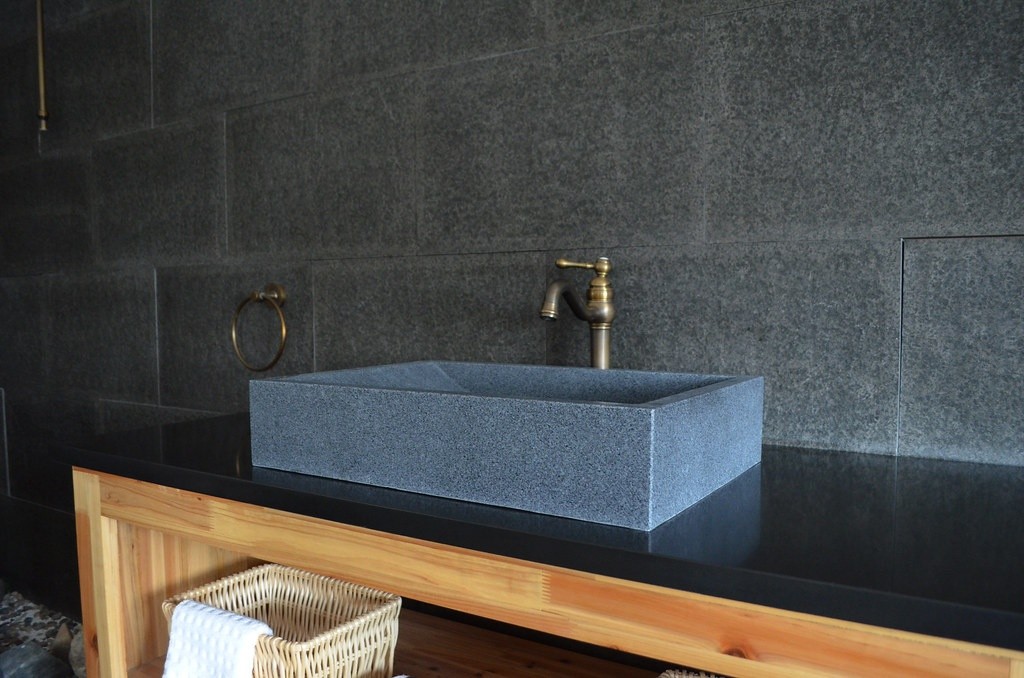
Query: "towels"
156 596 274 678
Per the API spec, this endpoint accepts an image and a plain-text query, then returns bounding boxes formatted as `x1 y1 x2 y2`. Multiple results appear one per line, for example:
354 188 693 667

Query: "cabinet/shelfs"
66 412 1024 676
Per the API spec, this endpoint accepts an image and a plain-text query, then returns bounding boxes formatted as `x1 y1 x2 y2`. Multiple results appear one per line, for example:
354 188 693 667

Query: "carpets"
0 590 87 678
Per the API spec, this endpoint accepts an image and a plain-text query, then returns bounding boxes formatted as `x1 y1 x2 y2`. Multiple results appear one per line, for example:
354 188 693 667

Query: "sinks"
245 355 767 533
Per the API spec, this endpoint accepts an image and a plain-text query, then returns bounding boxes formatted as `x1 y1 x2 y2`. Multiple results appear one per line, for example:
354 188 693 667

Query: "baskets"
161 563 402 678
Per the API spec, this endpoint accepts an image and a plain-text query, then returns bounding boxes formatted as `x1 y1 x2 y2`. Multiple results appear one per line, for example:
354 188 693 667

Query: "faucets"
537 256 617 325
